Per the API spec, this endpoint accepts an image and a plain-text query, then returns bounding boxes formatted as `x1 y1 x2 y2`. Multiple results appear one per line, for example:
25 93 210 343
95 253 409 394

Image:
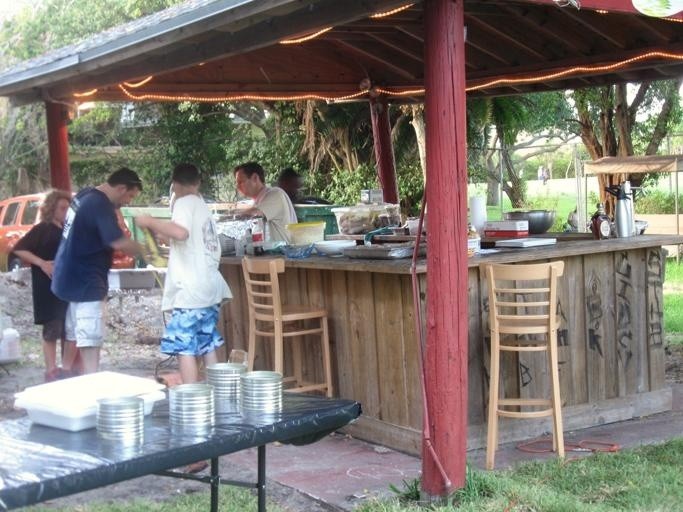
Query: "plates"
168 384 215 437
240 371 283 423
14 371 166 432
344 246 413 259
96 396 145 450
206 362 247 414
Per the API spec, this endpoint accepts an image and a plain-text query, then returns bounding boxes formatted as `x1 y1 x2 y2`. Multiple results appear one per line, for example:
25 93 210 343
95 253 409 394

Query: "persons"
133 163 233 381
232 164 299 240
14 193 78 382
52 168 141 374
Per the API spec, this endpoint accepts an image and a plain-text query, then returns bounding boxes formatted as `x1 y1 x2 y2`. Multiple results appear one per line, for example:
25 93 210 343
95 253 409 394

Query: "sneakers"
44 366 79 382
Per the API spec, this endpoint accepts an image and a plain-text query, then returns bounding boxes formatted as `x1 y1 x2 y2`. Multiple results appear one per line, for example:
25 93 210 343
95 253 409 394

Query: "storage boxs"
331 203 401 234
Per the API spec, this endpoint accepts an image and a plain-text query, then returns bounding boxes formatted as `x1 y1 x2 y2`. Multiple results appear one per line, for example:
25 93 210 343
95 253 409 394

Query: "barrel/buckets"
285 222 326 254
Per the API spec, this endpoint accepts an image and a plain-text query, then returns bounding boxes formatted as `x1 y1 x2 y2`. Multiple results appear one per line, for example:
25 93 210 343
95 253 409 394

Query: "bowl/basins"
279 243 315 259
314 240 357 258
503 210 556 234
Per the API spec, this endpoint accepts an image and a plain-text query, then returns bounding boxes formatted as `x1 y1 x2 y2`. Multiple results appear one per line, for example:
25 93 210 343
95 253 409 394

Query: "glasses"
127 180 143 186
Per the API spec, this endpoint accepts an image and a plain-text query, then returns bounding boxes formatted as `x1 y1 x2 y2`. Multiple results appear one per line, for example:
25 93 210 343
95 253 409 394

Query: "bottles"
251 220 263 256
466 226 481 257
591 203 612 239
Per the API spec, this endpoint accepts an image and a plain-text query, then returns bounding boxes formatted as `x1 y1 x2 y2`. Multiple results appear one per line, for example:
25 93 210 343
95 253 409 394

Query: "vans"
1 191 78 272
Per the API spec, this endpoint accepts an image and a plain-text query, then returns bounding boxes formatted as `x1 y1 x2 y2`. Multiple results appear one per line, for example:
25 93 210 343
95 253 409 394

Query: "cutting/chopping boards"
495 238 557 248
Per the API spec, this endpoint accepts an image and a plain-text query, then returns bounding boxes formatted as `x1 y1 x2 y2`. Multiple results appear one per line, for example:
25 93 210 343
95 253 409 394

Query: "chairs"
479 260 565 470
241 255 336 447
